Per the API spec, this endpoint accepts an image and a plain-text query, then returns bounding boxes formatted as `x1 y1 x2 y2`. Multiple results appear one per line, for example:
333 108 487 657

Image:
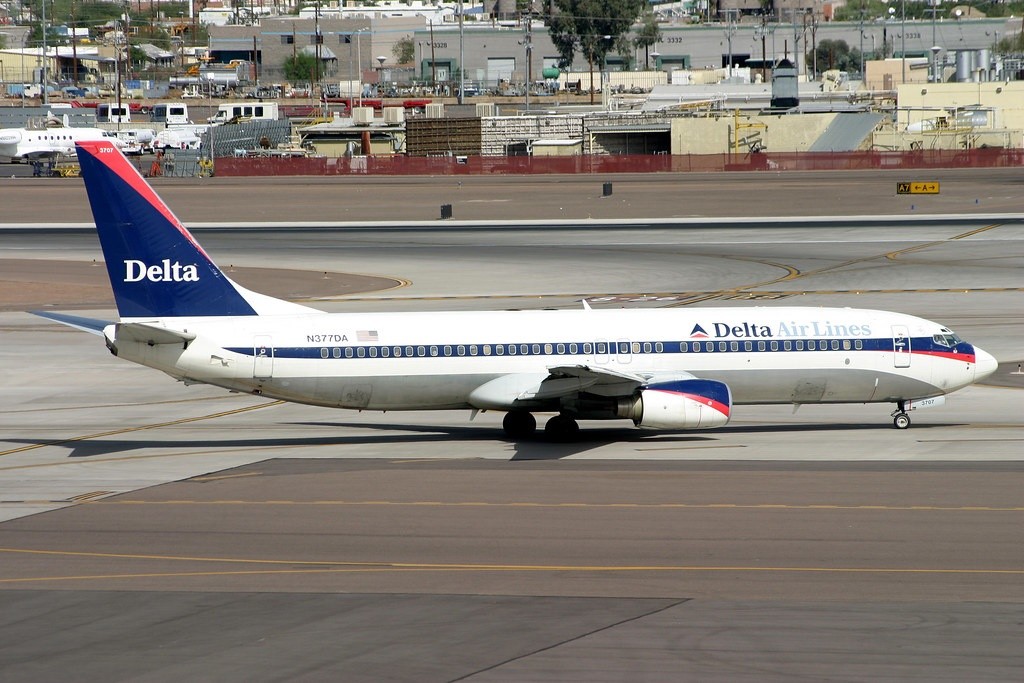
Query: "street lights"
930 43 946 82
355 24 368 107
886 5 904 63
955 8 968 50
589 33 613 107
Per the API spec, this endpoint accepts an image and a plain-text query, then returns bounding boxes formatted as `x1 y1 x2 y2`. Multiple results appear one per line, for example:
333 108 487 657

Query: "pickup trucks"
207 102 280 123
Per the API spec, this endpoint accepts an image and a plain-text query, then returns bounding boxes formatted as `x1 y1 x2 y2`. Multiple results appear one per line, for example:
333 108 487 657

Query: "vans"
150 102 192 128
97 102 132 123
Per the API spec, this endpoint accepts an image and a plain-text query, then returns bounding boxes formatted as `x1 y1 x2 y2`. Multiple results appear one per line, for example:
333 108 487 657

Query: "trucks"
205 102 282 124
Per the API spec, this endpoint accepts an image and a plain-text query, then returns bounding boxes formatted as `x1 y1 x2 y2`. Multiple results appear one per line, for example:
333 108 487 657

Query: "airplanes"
0 109 142 178
20 134 1001 445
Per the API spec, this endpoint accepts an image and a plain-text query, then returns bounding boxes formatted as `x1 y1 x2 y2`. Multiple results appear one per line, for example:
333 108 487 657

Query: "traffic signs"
896 181 941 195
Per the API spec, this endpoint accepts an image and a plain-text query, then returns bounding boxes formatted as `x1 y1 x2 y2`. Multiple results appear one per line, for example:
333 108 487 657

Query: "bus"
141 101 189 124
